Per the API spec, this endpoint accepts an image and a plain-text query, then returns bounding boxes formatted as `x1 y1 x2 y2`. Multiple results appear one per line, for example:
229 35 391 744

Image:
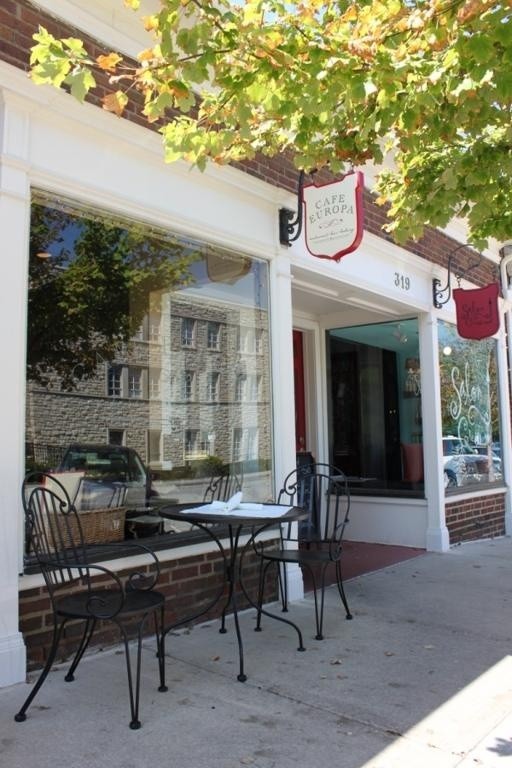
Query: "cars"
43 442 159 511
442 434 503 490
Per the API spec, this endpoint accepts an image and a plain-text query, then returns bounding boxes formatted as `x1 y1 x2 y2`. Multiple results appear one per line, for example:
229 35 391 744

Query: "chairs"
11 465 172 731
252 459 354 642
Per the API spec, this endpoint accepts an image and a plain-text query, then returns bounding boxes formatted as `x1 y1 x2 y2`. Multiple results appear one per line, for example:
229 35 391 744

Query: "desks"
156 497 314 682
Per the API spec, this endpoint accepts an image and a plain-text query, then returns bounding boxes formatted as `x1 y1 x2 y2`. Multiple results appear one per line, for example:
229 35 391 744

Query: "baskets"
47 507 126 547
